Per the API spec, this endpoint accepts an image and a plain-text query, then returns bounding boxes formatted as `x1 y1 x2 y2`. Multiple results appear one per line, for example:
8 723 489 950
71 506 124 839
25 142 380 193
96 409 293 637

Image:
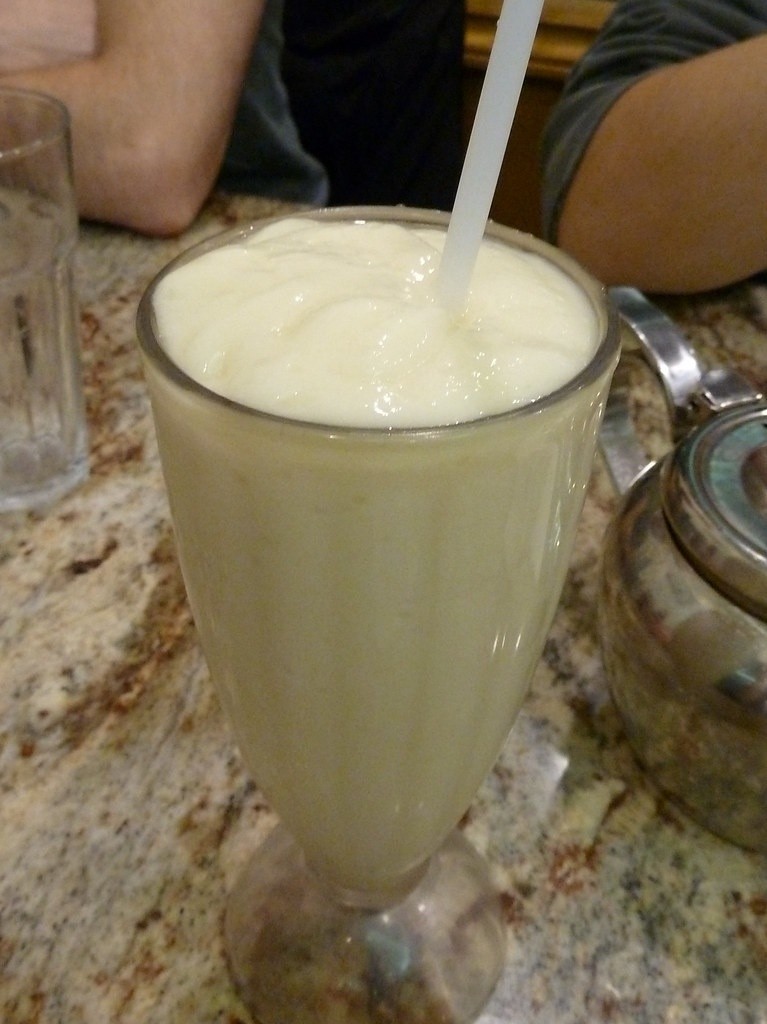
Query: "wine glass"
135 205 622 1024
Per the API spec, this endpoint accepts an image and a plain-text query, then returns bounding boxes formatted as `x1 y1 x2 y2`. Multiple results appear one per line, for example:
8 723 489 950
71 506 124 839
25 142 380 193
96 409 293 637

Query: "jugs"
602 283 767 853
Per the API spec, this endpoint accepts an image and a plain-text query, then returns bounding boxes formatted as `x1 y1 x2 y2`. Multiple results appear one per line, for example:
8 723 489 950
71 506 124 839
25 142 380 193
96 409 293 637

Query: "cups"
0 86 92 514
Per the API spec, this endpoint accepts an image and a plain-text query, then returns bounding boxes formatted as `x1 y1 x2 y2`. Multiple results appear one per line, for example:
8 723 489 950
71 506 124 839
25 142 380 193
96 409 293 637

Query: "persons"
538 0 767 295
0 0 466 237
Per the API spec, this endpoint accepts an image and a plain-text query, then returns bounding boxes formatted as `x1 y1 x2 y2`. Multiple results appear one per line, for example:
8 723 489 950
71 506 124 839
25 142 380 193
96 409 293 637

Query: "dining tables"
1 190 767 1024
